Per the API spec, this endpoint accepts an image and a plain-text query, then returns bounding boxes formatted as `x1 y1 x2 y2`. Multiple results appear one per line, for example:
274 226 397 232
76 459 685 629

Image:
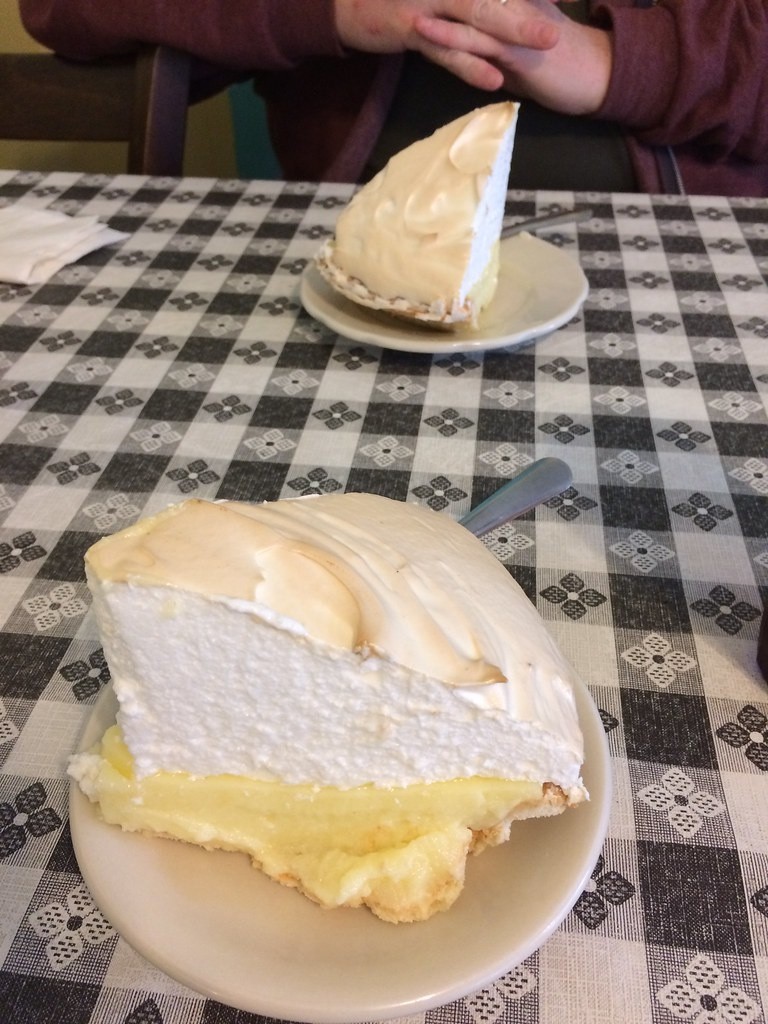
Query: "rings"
501 0 507 4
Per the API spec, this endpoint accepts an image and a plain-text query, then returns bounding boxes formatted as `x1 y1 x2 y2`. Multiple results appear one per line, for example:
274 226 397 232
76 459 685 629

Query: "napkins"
0 204 132 286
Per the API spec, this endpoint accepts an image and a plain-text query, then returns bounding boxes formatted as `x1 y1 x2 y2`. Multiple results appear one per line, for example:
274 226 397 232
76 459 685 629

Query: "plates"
68 654 610 1024
298 230 587 352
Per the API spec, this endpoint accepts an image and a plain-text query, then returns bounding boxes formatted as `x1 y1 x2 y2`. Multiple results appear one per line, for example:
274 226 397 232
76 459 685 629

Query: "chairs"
0 41 196 176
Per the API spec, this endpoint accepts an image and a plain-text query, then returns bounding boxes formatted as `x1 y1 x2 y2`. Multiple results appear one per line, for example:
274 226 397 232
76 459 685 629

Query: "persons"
15 0 768 200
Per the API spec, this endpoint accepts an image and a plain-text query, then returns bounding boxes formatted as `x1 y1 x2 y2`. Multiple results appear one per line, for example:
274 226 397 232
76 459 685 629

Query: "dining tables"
0 172 768 1024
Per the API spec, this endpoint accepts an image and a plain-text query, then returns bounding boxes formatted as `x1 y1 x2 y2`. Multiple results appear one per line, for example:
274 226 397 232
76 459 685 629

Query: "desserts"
317 102 520 327
65 497 591 921
